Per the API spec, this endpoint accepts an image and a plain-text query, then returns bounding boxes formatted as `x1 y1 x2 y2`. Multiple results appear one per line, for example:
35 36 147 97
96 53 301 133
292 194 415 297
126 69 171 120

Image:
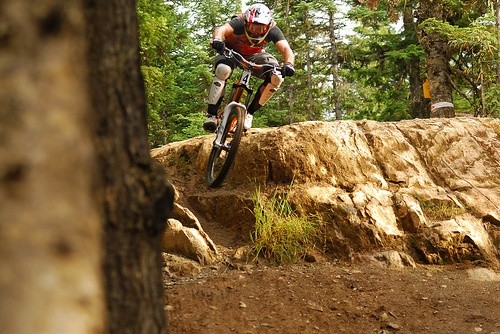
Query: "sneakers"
244 112 253 129
204 117 218 131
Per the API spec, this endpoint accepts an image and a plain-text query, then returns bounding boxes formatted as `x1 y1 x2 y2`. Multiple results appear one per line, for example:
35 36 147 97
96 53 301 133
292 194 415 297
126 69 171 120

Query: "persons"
202 4 296 133
423 77 431 107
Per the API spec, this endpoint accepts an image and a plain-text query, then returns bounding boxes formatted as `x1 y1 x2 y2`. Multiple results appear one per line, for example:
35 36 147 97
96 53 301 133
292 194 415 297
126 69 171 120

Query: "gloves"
280 62 295 78
212 39 225 55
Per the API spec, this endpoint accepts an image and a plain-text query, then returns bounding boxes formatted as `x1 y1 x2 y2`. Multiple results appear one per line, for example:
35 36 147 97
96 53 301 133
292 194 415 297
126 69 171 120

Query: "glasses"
246 21 269 34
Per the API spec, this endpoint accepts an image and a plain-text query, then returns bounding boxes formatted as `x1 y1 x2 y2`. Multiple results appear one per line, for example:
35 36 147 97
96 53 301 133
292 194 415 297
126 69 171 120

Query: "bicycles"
206 41 286 188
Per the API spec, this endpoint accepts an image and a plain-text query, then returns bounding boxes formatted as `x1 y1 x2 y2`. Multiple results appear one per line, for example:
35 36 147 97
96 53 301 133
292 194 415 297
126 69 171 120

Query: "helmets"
242 3 275 45
